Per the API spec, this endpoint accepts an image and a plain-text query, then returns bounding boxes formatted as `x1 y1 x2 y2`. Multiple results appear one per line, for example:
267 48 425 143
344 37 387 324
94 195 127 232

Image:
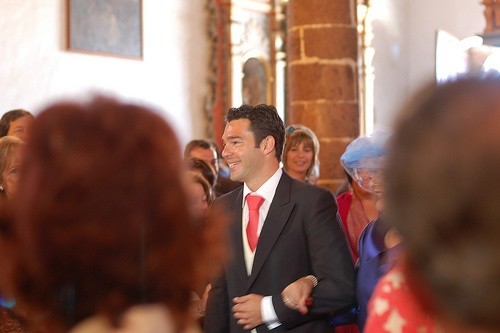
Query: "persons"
204 104 355 333
282 125 320 183
0 94 237 333
283 131 401 333
363 74 500 333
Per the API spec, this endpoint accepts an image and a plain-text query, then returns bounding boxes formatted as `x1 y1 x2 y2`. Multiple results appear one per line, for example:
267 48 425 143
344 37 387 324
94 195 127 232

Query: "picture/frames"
67 0 144 60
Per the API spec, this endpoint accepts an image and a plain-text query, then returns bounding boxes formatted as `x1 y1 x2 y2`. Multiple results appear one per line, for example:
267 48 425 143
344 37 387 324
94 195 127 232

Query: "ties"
245 193 266 252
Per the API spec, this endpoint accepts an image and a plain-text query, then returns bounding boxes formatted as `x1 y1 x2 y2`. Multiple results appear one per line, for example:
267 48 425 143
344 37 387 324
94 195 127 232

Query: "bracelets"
306 275 318 286
